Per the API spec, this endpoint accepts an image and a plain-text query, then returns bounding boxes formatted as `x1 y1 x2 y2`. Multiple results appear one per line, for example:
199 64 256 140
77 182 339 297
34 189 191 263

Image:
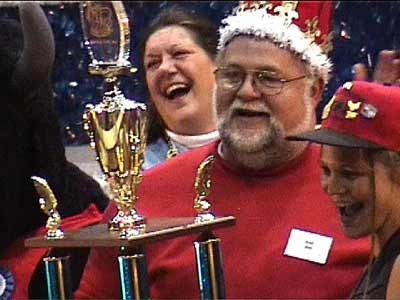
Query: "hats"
217 0 333 83
284 81 400 152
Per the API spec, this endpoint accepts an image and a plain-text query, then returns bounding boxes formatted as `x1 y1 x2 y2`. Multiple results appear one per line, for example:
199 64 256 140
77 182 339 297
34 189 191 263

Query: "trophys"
24 0 235 300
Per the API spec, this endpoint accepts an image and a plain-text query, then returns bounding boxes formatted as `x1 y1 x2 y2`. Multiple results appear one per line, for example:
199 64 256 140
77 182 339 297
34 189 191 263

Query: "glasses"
213 69 307 96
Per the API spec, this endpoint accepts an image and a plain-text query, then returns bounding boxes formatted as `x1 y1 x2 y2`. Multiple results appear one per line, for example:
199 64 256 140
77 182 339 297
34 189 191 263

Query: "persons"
142 11 222 172
0 1 64 245
287 80 400 300
74 0 400 300
